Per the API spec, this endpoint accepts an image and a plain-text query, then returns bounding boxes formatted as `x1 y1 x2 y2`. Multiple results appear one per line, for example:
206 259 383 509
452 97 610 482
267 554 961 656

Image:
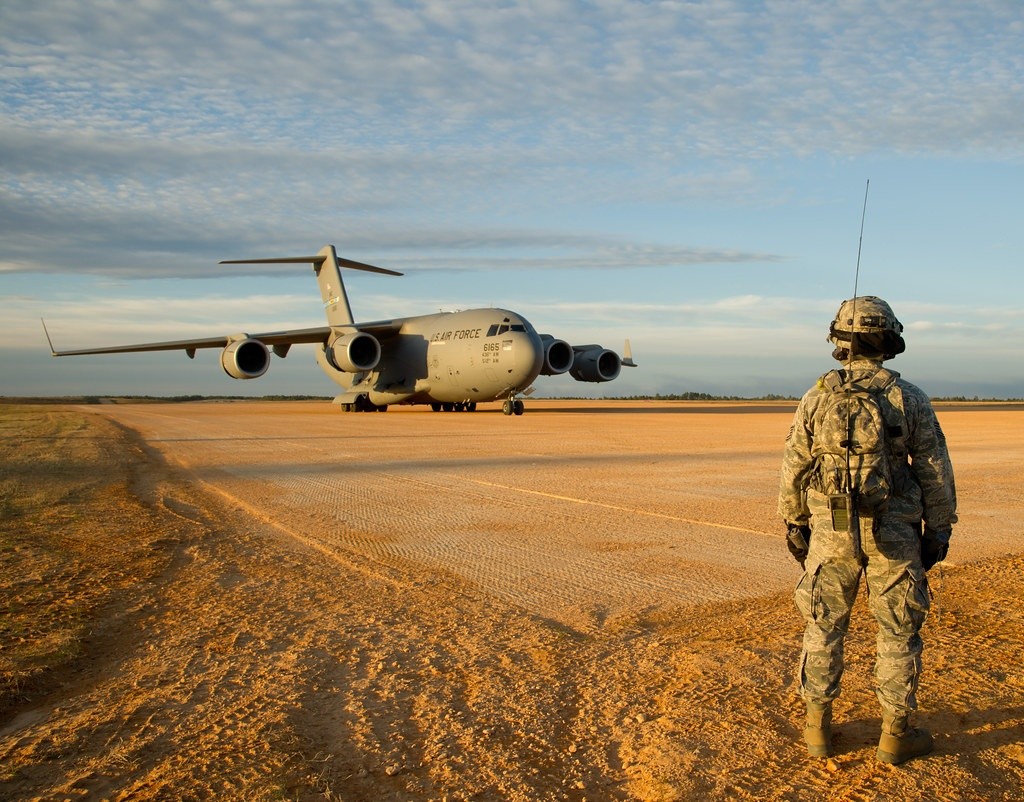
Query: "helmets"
829 295 902 352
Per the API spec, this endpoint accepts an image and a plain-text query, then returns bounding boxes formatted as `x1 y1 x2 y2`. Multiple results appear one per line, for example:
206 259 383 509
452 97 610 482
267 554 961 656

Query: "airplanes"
41 241 639 415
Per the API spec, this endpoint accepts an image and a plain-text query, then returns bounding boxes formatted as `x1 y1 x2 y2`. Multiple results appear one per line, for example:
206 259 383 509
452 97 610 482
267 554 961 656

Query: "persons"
777 295 959 764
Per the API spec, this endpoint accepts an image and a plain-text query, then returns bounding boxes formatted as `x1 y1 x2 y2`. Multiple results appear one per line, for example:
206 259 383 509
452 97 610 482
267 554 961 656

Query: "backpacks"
818 369 900 503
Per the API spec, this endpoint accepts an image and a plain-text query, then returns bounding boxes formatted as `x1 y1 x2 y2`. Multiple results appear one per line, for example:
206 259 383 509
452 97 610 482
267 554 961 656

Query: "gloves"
921 528 950 572
786 527 812 563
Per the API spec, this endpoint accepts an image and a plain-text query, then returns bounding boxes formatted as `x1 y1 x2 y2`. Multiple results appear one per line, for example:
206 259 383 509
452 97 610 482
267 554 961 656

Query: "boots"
804 702 834 755
876 709 933 765
